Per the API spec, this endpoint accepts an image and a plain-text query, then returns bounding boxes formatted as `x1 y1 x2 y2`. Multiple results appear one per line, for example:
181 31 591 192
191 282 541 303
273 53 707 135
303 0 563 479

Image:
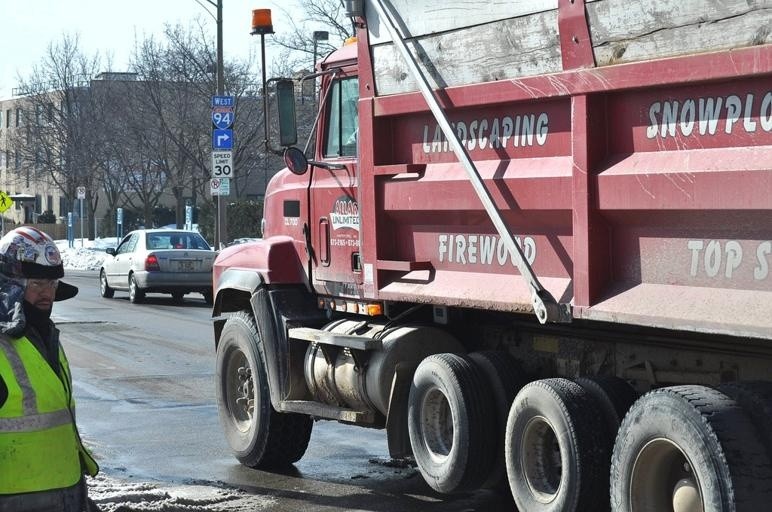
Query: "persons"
0 225 100 512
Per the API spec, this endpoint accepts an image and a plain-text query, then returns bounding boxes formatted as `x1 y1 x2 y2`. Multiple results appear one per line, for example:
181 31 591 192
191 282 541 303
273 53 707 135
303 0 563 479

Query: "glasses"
28 279 59 293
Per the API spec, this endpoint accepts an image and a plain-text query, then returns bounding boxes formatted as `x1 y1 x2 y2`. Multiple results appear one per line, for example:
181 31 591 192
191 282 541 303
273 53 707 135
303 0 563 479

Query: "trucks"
206 0 772 511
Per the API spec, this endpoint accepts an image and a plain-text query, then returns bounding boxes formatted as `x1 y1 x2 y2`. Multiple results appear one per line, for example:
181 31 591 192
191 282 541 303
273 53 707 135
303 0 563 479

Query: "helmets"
0 226 78 336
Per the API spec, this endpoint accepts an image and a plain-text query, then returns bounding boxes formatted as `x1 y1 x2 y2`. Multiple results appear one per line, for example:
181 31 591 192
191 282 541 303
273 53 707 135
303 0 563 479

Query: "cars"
97 228 218 306
